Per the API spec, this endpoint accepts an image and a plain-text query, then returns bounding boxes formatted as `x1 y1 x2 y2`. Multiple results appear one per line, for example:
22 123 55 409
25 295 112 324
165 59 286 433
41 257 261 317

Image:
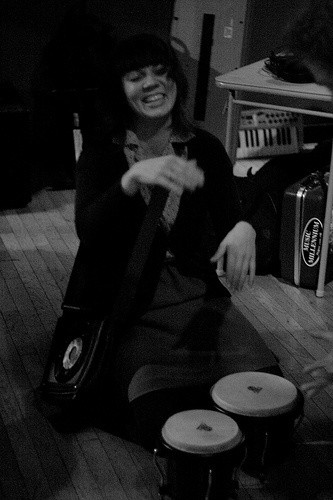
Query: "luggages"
276 170 333 292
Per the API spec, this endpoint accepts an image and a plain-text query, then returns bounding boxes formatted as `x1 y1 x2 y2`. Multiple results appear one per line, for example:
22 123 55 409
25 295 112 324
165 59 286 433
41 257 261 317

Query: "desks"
214 48 333 299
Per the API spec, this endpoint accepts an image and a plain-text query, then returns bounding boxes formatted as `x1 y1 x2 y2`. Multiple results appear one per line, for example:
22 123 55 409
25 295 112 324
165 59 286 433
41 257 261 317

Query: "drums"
152 408 246 499
209 369 306 469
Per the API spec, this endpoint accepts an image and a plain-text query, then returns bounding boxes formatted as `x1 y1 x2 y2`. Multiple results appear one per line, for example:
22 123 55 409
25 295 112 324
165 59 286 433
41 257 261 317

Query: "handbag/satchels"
40 306 113 405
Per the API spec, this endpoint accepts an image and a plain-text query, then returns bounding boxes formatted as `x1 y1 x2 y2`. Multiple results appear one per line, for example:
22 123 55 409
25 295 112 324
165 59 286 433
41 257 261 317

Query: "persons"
38 31 301 483
